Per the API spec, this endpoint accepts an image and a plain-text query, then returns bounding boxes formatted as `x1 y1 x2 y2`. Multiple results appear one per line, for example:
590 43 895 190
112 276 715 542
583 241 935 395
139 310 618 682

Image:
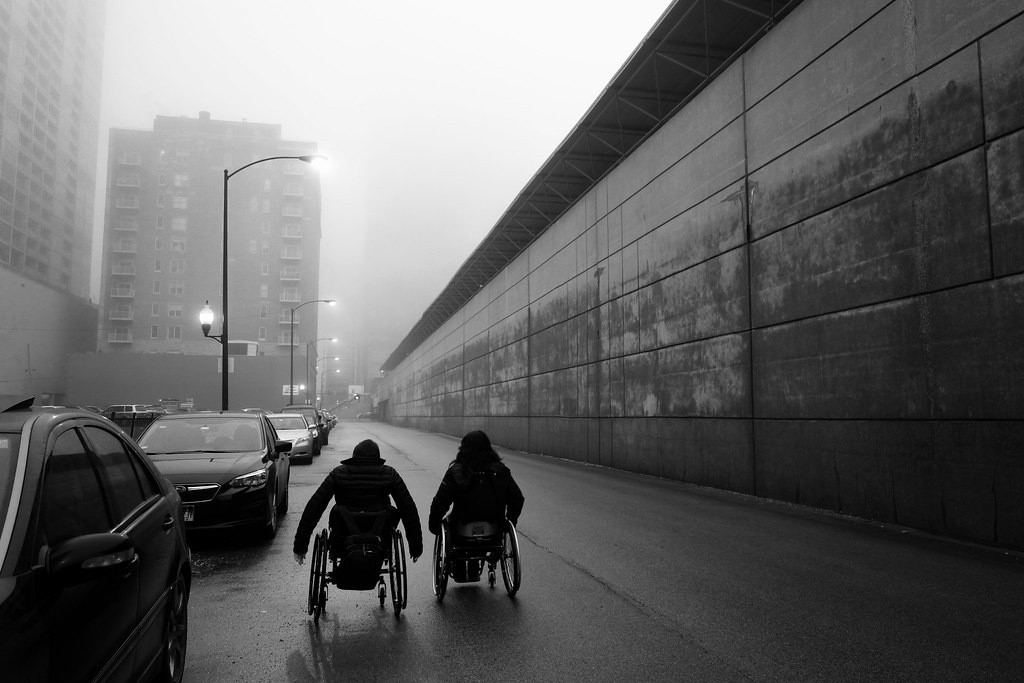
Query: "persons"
427 430 524 556
292 438 426 564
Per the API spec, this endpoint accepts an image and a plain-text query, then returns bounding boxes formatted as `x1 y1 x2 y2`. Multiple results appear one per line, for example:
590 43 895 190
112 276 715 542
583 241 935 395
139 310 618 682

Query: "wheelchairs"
430 513 522 603
307 510 408 621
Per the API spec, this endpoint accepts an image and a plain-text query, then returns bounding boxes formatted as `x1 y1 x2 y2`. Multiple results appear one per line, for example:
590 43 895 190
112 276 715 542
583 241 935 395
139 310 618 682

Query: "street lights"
305 337 340 407
290 300 338 405
199 153 330 412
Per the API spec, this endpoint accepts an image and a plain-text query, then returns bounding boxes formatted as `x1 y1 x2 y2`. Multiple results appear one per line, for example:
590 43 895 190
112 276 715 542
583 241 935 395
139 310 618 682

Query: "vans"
279 405 324 456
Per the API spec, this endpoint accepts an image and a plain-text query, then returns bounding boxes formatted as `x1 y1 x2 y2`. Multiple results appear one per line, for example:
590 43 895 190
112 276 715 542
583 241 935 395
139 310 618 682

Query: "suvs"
102 404 147 417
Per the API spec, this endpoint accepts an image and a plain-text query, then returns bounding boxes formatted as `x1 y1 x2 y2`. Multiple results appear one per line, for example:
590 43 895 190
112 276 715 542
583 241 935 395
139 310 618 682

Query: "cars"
266 413 316 463
0 395 190 683
145 404 191 416
135 411 292 540
317 409 338 444
